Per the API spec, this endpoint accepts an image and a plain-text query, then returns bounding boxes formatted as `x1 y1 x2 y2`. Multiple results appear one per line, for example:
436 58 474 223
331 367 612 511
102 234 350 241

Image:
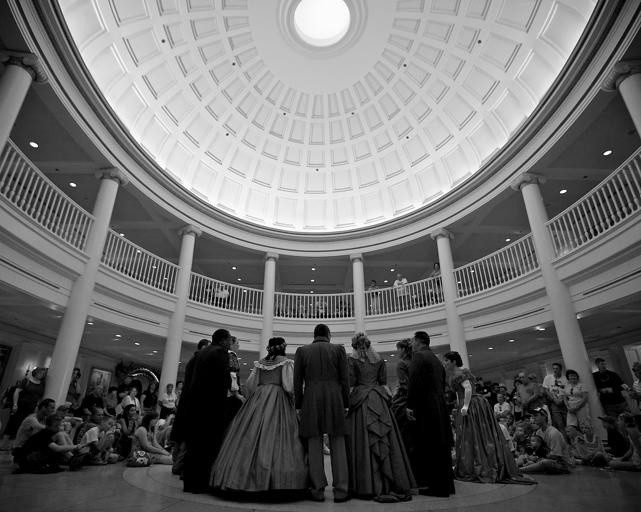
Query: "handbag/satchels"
127 449 154 467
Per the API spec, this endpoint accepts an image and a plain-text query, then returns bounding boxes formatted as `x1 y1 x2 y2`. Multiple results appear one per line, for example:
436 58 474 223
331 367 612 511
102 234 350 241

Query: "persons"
213 285 231 308
334 298 352 318
388 336 412 430
368 278 382 315
292 322 354 504
227 335 247 427
209 335 310 502
345 329 418 503
275 298 290 318
474 358 641 476
313 298 328 317
169 337 213 475
180 327 234 494
393 273 412 313
7 367 178 476
294 298 307 318
429 262 445 304
441 350 507 485
406 330 457 500
